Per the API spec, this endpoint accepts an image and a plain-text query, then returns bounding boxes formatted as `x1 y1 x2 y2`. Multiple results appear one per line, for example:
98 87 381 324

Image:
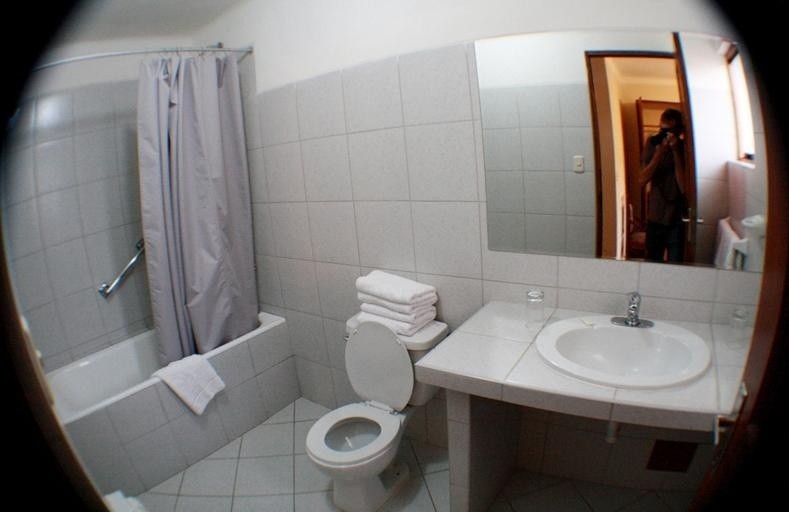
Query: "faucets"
625 290 642 325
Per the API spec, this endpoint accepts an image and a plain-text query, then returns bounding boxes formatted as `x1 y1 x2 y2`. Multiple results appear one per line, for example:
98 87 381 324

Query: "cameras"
660 129 670 137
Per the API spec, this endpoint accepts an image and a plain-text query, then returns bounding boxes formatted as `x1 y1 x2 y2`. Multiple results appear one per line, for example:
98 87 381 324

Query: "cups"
524 289 545 328
727 309 748 350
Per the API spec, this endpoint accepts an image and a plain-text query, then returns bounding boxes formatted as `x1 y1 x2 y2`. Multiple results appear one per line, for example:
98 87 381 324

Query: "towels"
151 353 226 415
712 219 744 272
356 270 440 338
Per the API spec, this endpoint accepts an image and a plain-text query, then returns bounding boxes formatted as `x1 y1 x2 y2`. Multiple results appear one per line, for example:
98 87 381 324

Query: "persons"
639 108 685 264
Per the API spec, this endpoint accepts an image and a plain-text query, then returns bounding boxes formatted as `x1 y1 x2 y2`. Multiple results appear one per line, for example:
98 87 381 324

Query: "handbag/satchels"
647 186 674 226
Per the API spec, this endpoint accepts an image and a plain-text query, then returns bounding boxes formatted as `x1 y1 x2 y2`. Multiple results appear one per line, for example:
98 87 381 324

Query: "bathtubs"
44 310 287 430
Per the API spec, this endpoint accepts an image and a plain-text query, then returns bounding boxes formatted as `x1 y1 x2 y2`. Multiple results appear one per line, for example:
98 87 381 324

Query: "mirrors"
474 31 749 271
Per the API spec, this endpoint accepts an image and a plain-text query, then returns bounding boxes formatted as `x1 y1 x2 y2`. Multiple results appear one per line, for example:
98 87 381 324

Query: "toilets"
303 311 450 512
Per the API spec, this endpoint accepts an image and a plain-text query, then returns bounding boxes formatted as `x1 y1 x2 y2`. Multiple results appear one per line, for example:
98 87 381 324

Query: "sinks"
534 315 712 390
